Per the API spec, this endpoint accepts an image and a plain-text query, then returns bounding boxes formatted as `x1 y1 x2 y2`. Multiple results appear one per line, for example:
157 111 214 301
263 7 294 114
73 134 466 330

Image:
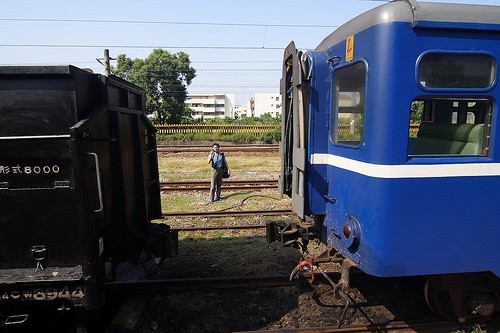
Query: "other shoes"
210 199 215 203
215 198 222 201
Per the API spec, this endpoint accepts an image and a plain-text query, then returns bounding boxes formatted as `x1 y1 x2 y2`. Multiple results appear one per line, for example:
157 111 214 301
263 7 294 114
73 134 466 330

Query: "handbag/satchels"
222 163 230 178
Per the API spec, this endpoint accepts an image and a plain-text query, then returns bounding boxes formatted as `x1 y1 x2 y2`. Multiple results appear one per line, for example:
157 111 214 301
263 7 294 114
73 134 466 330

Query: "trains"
263 2 499 326
1 49 183 333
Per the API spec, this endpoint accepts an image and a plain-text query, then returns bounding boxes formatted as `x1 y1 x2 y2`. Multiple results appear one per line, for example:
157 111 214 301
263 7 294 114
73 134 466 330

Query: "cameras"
212 163 217 168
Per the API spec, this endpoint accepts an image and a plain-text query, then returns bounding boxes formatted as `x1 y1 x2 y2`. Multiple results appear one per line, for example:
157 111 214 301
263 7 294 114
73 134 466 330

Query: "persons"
207 143 230 204
